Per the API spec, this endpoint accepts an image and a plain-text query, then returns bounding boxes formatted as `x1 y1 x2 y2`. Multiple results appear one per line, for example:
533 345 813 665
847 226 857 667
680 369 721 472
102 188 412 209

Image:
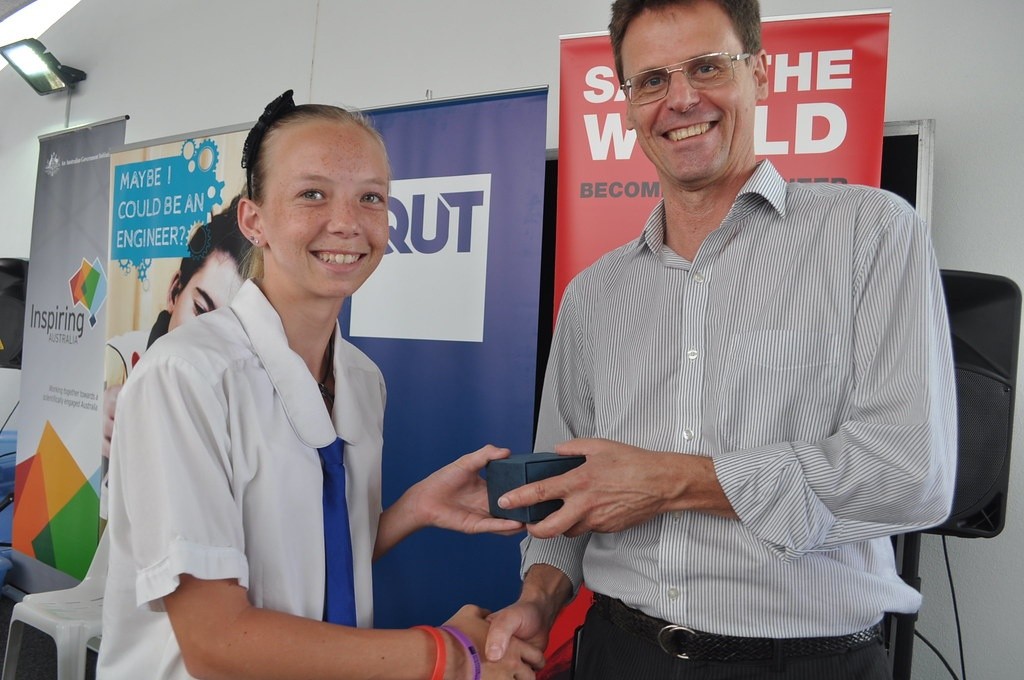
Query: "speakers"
0 257 28 370
918 269 1022 539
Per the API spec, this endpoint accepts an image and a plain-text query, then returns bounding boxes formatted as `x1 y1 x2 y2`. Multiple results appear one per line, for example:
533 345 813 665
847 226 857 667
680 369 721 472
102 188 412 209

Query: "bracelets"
440 626 482 680
411 625 447 680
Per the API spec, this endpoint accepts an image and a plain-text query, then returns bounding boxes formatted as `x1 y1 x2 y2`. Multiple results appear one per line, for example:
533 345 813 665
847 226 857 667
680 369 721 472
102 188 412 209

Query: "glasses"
620 52 751 105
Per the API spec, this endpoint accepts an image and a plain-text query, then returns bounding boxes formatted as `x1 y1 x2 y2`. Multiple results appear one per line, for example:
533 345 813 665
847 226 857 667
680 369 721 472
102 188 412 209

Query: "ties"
318 437 356 629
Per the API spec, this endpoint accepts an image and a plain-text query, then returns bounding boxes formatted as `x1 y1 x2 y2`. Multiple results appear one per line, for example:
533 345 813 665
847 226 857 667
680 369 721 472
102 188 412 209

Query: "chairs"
1 520 109 680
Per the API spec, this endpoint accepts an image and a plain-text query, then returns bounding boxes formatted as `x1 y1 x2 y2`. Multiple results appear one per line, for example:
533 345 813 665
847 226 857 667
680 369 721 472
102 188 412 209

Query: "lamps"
0 38 86 97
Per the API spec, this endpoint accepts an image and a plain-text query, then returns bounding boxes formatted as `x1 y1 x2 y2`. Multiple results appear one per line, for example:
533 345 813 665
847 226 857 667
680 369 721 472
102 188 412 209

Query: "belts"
602 598 880 660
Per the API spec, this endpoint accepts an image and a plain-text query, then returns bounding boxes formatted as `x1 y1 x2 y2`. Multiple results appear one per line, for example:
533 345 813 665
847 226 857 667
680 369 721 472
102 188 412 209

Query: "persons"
101 195 253 459
98 90 547 680
484 0 959 680
